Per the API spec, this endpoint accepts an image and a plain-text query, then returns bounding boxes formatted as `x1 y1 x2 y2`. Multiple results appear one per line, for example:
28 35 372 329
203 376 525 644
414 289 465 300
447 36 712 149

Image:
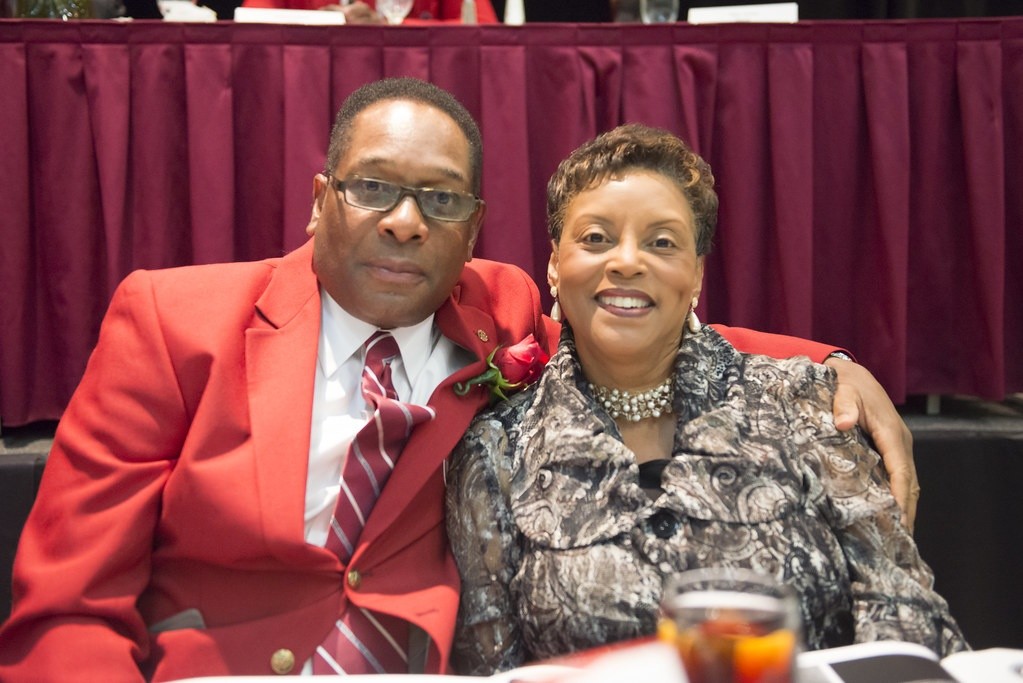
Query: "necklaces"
582 370 679 421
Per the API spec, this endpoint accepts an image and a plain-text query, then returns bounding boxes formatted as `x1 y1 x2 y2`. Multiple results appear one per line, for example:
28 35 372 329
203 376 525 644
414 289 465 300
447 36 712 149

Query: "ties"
313 330 436 675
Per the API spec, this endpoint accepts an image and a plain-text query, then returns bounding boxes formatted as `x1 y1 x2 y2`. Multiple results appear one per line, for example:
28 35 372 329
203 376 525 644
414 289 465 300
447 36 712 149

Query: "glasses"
325 169 480 223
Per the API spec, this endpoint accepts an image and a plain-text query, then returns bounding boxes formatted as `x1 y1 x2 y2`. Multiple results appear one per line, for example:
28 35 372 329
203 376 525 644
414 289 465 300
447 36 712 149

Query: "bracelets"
831 352 852 361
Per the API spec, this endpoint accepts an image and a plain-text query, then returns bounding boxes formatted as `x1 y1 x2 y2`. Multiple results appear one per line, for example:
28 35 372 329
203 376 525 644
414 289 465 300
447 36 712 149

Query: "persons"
0 76 921 683
243 1 503 26
446 121 973 675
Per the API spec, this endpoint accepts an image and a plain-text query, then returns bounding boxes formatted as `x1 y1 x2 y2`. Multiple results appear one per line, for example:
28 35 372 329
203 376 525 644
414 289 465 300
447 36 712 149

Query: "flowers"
453 334 551 411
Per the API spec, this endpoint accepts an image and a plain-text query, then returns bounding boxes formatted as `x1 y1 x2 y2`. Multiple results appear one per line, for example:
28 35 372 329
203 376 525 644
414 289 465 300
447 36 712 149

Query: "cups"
640 0 679 24
663 567 801 683
